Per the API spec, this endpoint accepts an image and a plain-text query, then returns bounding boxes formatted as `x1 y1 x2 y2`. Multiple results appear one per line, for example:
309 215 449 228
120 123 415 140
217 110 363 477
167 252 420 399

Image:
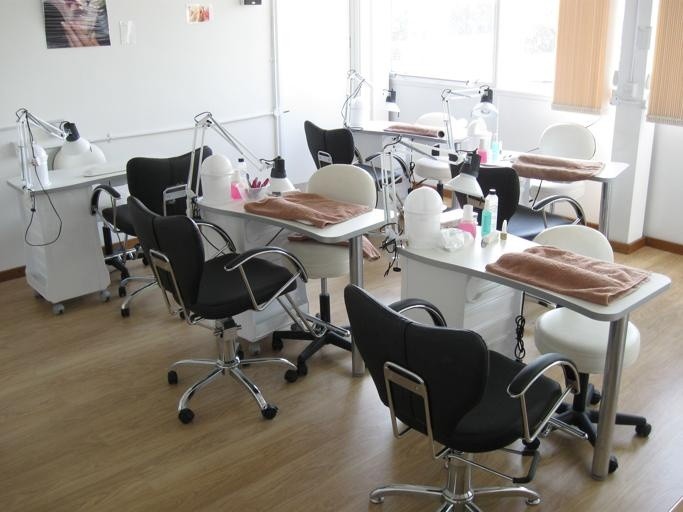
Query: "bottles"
236 157 246 183
483 189 498 236
476 137 499 163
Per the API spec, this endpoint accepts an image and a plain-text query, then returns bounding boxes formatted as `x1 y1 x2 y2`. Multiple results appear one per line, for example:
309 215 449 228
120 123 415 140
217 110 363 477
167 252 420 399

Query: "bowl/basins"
234 182 271 204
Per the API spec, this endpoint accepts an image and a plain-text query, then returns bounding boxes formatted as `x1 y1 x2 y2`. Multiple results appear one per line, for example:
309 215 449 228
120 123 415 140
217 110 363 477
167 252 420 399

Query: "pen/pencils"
246 173 270 197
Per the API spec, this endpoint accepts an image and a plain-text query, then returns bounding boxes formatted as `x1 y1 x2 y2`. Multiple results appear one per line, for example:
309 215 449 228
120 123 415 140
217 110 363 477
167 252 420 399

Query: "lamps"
340 69 399 128
379 133 488 255
183 110 295 221
441 84 497 153
15 107 107 189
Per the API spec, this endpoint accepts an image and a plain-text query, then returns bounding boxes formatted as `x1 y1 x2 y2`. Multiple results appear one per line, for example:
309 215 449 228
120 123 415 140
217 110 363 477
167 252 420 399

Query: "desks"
456 146 631 247
395 224 672 481
6 162 128 314
344 122 450 162
195 187 403 379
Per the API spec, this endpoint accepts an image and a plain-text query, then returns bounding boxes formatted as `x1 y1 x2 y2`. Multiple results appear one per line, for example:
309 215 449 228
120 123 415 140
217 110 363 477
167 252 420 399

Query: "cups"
462 204 473 222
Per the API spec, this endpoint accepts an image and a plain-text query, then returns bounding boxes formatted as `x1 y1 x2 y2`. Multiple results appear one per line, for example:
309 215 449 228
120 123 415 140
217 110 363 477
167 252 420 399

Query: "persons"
42 1 110 50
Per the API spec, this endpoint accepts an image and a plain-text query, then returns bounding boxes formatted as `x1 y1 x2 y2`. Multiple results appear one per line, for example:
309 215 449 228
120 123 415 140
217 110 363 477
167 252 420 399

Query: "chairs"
449 162 588 239
529 122 600 200
92 144 215 320
268 162 378 386
127 195 309 425
531 224 653 474
343 283 589 512
302 120 412 222
